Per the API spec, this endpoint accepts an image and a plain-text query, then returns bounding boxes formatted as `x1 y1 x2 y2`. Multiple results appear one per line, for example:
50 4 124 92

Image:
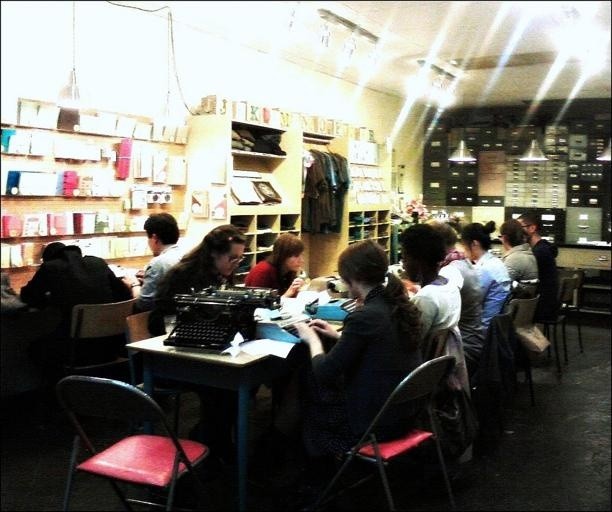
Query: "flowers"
406 194 427 219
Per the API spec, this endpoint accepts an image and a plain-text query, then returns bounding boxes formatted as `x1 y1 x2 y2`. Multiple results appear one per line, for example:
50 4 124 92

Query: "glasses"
229 258 243 264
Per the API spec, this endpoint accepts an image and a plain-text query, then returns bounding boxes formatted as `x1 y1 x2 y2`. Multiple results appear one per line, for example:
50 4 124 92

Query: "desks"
456 242 611 315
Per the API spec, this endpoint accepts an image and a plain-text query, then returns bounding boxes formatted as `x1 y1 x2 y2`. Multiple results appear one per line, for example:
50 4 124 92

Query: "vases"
411 212 418 223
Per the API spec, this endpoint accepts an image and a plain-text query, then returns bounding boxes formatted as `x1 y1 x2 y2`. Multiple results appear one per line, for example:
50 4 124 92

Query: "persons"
243 233 304 298
18 242 131 416
147 224 247 479
284 211 561 512
120 211 191 310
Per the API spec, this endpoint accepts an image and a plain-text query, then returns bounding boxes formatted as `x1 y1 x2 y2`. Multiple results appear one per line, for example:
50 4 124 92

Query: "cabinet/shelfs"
0 97 188 295
421 98 611 252
310 138 391 278
188 115 302 277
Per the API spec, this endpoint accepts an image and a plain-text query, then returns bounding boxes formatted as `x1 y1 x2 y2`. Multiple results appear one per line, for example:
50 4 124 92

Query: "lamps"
596 100 612 162
58 1 192 127
518 101 549 161
411 57 466 104
289 1 381 65
448 106 477 162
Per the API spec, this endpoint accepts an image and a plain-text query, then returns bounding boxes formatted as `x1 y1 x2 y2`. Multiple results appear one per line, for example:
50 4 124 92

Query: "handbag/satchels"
432 379 483 458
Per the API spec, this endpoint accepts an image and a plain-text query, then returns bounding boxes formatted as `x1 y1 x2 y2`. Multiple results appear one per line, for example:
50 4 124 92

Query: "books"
192 97 385 253
1 96 192 270
